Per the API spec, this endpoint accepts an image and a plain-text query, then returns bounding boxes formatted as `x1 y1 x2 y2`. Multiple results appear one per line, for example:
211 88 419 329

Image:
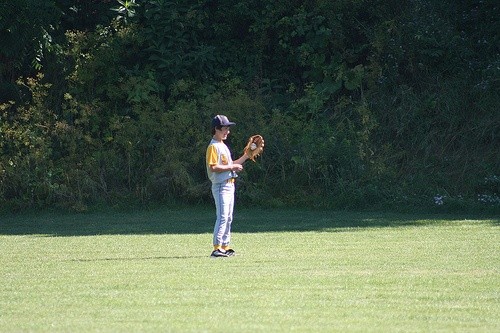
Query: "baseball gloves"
244 133 266 161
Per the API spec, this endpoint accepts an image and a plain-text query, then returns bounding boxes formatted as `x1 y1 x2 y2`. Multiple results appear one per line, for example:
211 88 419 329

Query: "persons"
206 115 264 257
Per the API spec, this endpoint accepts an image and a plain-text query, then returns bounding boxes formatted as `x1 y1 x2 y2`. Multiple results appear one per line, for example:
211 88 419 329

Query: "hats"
210 115 236 127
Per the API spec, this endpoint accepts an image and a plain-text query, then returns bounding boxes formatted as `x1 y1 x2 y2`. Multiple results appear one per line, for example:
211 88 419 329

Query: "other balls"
250 143 258 151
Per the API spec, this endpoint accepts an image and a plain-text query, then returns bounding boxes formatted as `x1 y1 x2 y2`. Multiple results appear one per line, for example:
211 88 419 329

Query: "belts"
224 178 235 183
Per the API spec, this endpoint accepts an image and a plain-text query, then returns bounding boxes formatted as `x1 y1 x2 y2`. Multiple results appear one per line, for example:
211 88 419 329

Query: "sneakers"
210 249 235 257
